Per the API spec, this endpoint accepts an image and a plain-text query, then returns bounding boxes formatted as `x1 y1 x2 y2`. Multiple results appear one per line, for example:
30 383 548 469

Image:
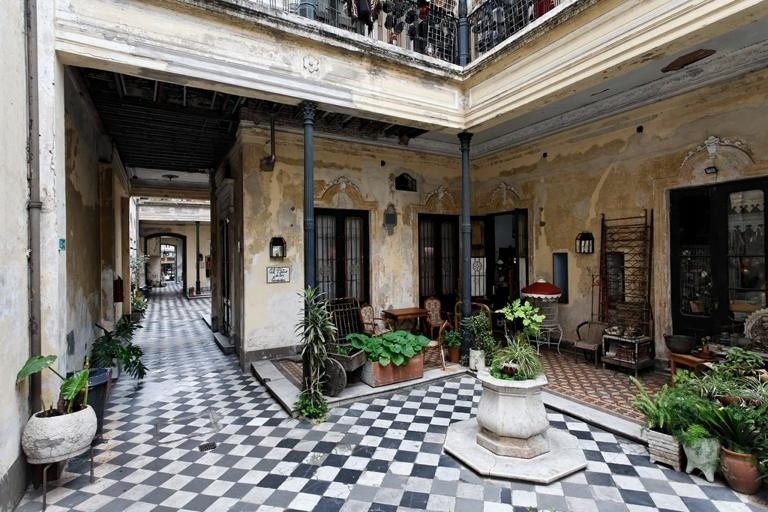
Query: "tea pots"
604 325 624 336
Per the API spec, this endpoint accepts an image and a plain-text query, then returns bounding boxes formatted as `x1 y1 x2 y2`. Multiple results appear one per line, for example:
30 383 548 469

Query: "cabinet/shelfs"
601 331 653 374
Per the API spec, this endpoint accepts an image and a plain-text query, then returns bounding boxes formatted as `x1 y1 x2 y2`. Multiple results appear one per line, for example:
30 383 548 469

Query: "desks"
381 307 429 337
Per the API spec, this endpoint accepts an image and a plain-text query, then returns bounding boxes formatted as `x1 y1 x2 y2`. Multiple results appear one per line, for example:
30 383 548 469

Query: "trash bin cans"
64 369 109 446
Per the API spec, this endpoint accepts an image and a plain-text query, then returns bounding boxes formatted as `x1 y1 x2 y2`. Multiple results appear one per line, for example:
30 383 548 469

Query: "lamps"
704 165 718 175
574 233 594 253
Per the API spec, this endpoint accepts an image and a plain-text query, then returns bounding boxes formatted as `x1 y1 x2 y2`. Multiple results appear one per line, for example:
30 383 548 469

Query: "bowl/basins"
662 333 693 353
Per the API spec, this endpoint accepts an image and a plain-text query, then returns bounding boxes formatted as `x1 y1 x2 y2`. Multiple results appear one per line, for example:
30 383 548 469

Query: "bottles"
700 331 738 352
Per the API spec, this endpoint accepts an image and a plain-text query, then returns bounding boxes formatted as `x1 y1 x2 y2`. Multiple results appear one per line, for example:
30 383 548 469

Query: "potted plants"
491 299 548 377
441 330 463 363
462 312 494 373
346 331 426 390
627 344 767 497
17 254 153 512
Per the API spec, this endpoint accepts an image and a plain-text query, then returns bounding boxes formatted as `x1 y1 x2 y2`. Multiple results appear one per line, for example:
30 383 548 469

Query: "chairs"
532 300 563 355
424 296 454 341
453 301 492 337
572 319 606 369
360 303 395 338
422 320 446 371
